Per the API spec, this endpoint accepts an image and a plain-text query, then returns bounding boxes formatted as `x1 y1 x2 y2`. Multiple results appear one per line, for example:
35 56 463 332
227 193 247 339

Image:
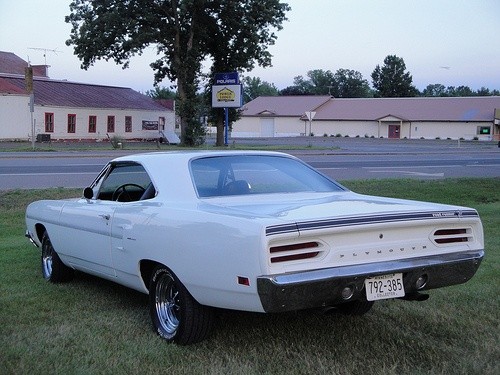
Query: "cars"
25 150 484 344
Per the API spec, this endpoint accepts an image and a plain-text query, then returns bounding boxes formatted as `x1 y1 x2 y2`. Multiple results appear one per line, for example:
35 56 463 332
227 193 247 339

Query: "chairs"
223 180 251 195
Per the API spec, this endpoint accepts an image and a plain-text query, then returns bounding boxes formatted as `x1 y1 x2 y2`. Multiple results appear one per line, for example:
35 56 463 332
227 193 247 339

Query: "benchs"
35 133 54 148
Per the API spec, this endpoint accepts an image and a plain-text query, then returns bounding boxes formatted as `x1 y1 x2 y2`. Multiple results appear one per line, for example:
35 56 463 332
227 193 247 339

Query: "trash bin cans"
37 134 50 143
